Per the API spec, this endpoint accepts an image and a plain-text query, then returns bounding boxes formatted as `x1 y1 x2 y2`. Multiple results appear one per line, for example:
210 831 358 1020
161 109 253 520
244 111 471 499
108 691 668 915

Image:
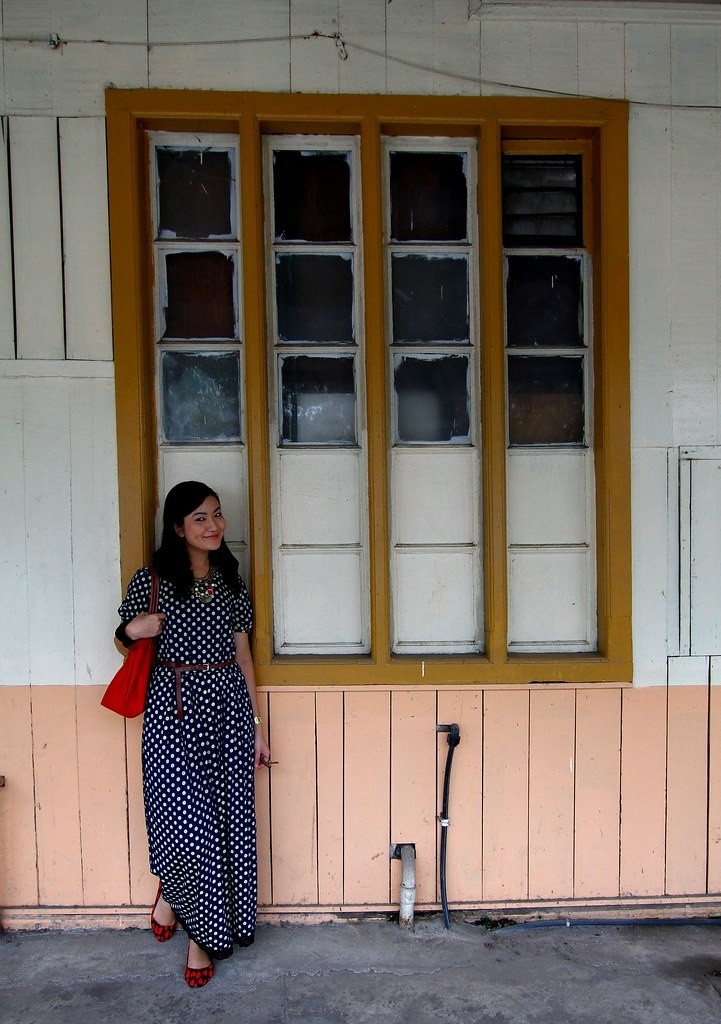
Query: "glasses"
260 753 279 769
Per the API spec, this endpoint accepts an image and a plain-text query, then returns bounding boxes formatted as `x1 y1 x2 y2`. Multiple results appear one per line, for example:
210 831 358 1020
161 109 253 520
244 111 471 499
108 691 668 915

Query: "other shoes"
184 945 214 988
151 879 178 943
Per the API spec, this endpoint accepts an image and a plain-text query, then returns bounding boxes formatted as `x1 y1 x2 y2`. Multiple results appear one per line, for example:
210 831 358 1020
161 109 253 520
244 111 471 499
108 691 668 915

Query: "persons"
112 481 271 988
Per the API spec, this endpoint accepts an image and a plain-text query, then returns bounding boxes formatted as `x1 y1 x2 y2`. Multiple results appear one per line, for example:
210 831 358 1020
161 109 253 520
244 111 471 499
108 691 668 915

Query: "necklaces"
190 566 216 604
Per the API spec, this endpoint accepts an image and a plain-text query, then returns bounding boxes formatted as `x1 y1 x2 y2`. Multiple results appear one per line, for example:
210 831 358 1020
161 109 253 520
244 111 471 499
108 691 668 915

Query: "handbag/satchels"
101 576 159 719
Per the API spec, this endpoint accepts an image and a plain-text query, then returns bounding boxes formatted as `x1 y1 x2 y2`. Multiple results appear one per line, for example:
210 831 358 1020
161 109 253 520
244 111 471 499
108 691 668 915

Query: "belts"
156 659 236 719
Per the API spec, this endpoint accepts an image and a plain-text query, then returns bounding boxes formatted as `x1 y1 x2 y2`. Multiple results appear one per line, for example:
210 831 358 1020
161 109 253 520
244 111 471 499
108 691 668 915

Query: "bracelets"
253 717 261 725
114 620 139 648
